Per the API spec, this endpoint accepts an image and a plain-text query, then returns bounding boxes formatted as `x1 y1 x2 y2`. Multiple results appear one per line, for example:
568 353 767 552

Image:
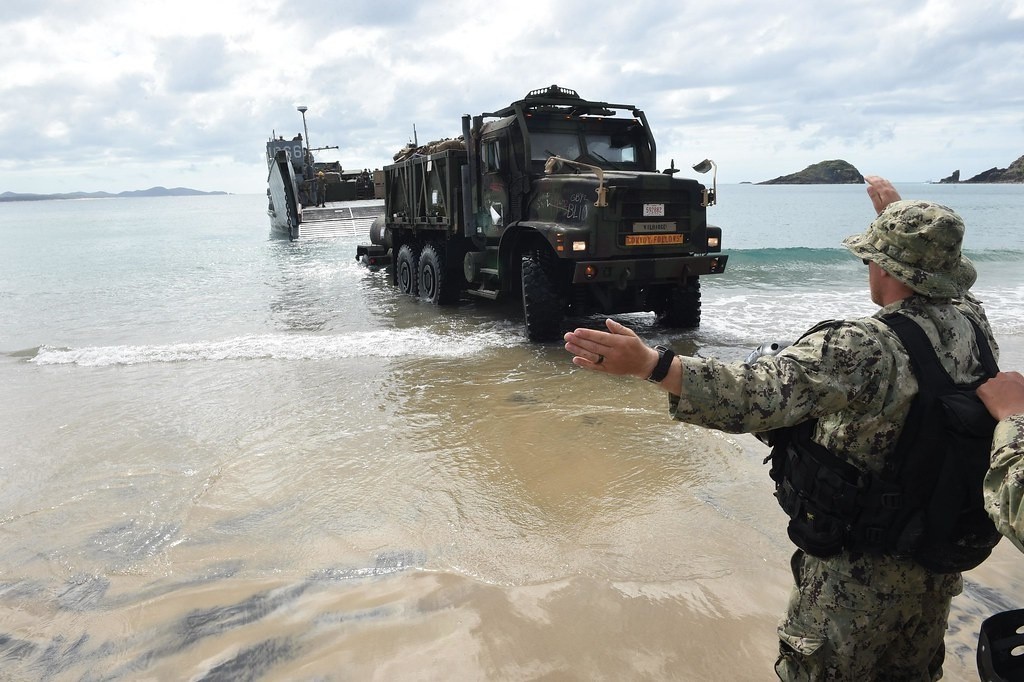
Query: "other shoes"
316 202 319 207
322 202 325 207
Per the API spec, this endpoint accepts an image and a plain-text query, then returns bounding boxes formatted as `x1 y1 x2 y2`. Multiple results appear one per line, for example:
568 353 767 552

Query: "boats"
264 106 385 242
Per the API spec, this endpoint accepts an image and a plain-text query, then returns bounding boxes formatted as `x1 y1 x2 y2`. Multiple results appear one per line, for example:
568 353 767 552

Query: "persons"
976 370 1024 553
361 169 370 188
560 175 1003 682
305 170 328 207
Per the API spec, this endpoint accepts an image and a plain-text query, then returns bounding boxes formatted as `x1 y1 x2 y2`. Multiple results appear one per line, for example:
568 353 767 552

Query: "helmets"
744 341 789 448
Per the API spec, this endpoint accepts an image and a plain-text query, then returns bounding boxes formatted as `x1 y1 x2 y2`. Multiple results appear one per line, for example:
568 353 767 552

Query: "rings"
595 354 603 365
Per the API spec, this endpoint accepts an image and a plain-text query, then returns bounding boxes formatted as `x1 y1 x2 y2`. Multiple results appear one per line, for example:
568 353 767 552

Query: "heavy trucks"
354 84 729 346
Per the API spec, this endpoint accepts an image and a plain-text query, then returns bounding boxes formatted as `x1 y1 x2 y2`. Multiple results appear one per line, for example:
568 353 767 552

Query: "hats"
316 171 324 175
839 197 979 298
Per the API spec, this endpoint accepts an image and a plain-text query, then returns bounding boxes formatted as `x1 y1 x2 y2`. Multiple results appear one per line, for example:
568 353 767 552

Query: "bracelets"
643 343 675 384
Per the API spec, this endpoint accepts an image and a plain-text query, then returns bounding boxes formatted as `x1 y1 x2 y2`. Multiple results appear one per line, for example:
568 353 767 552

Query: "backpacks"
867 308 1012 574
